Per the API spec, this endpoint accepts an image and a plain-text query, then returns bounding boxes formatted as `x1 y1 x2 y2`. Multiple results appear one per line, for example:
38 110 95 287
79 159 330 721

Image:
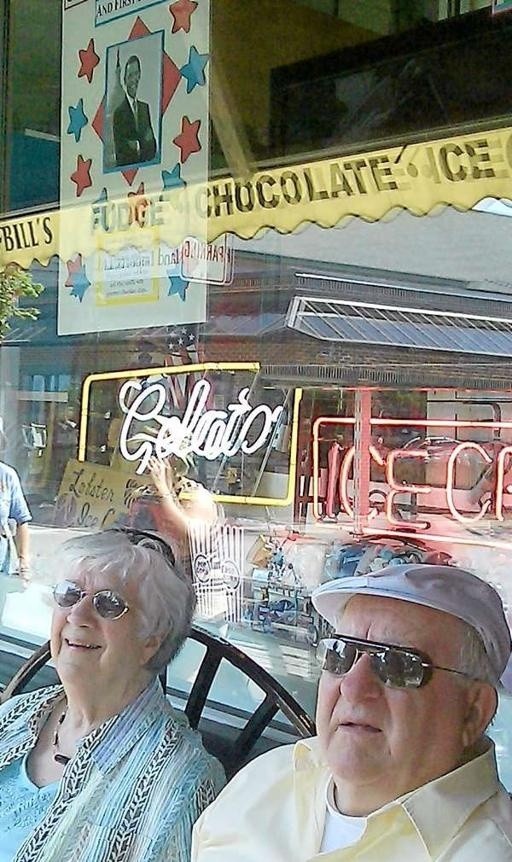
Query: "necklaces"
48 702 87 767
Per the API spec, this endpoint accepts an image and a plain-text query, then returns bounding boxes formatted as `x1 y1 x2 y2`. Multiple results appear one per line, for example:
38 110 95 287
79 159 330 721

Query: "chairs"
0 622 319 774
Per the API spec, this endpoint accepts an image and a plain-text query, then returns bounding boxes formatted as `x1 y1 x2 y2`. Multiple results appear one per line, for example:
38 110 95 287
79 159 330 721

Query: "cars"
373 437 391 461
476 441 511 455
392 434 465 465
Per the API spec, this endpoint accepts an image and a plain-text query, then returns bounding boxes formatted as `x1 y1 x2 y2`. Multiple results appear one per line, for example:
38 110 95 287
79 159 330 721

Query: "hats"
310 563 511 680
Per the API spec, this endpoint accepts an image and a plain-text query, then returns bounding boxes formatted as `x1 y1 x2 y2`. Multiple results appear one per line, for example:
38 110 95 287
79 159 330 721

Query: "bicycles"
195 538 242 591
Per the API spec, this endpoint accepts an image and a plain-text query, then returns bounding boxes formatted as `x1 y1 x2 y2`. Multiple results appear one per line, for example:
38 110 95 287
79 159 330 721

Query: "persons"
110 46 165 163
0 527 227 862
1 414 37 609
130 441 223 555
188 563 511 862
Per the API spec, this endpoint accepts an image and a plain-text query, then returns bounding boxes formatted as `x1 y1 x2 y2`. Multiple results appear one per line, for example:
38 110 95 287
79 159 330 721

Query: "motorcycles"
244 506 325 648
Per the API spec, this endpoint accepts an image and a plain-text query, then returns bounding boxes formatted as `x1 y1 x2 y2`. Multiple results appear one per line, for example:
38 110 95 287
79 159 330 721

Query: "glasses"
48 578 131 622
315 634 477 690
